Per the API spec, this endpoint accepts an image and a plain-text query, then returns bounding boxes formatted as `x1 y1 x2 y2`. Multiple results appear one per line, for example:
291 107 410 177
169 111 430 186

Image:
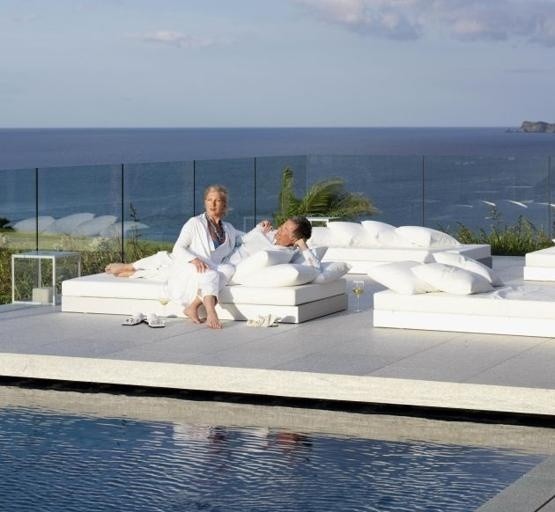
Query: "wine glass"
158 293 171 322
348 278 366 314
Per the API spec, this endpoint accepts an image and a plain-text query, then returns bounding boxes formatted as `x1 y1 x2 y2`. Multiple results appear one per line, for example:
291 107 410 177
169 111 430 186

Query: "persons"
168 182 242 328
171 425 224 464
237 431 313 476
104 215 321 288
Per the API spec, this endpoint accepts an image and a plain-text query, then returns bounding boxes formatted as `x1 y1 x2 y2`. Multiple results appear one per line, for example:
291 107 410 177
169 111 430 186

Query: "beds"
373 282 555 337
61 272 348 324
523 246 555 280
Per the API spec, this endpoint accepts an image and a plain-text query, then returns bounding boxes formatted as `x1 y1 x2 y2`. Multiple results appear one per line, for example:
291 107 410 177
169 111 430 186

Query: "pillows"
231 217 505 296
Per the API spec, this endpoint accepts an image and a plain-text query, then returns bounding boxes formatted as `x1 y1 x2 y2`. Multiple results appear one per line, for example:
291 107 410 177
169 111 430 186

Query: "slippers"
121 312 165 327
